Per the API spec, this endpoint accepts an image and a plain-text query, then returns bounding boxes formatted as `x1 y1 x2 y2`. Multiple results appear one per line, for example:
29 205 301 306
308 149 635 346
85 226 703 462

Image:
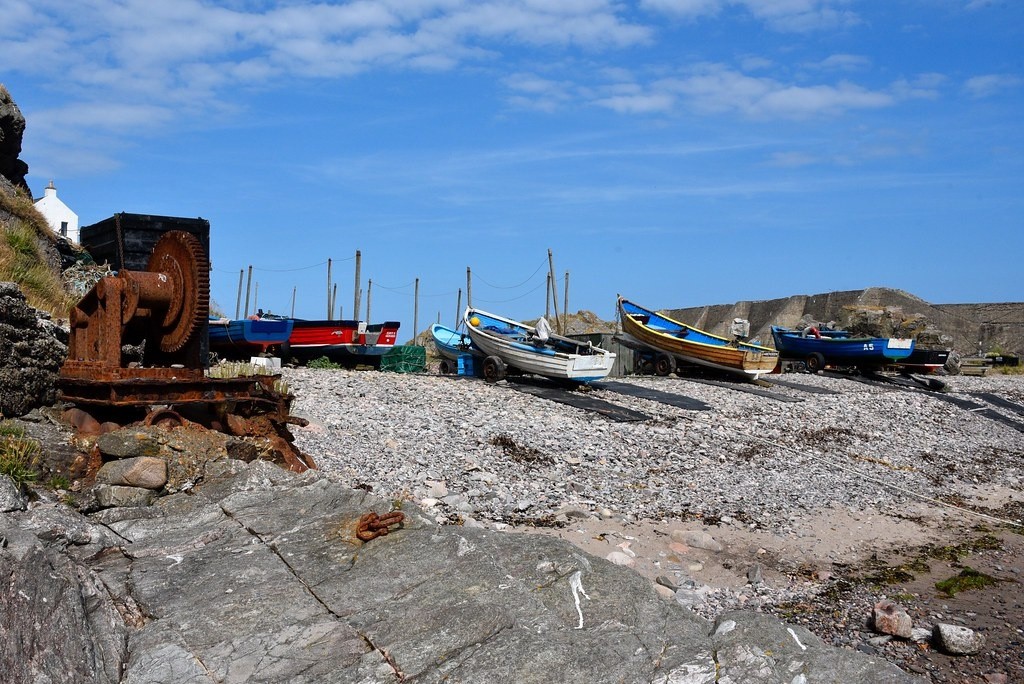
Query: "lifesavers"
802 327 821 339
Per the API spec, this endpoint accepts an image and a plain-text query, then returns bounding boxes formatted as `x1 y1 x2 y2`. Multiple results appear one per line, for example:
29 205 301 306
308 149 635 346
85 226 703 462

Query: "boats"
851 331 951 376
770 325 917 368
430 322 481 362
256 308 401 360
208 315 295 356
615 292 780 376
463 306 617 383
